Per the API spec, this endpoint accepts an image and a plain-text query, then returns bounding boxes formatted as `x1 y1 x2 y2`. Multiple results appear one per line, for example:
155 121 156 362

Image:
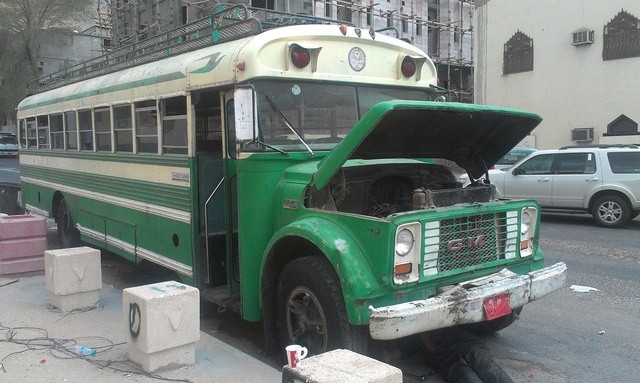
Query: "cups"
285 344 308 369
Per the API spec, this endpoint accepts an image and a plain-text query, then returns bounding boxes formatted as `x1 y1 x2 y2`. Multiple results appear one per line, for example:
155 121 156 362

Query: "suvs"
479 142 640 228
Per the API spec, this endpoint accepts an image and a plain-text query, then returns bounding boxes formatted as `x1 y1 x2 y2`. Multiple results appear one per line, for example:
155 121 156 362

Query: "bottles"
73 345 97 356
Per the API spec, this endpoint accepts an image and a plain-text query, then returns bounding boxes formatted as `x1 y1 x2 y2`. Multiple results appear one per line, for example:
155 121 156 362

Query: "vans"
488 145 538 171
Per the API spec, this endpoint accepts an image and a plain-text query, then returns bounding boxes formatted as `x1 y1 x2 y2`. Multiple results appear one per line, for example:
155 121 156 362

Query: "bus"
14 4 568 368
